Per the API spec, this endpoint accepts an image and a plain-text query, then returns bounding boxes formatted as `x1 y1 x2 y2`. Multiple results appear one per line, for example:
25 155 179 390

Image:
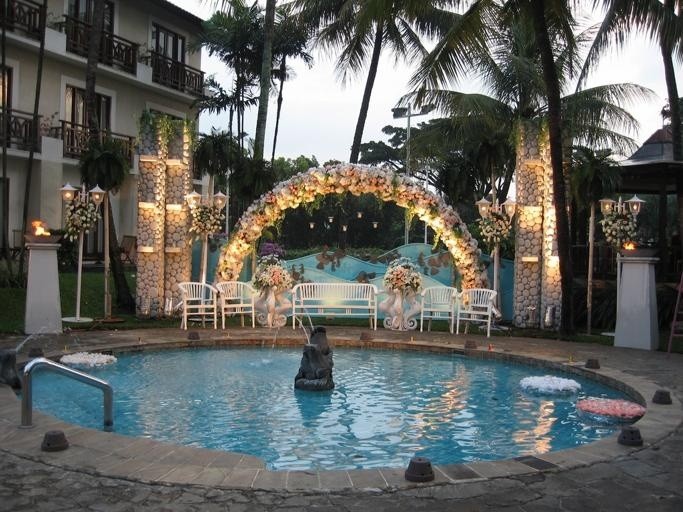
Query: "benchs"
291 283 379 330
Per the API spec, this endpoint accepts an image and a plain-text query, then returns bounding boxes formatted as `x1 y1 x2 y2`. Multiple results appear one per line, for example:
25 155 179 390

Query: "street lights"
476 158 517 323
390 98 433 244
60 178 103 322
183 187 228 312
599 192 646 317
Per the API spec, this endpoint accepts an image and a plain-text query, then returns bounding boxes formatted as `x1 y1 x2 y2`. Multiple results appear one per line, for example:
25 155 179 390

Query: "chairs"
118 235 136 265
455 289 497 338
419 287 457 334
178 282 218 331
216 280 256 329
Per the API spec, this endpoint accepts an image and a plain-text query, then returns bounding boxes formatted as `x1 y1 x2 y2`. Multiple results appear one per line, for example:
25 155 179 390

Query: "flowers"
212 162 487 292
478 218 512 244
600 211 637 247
189 206 226 244
64 197 101 241
